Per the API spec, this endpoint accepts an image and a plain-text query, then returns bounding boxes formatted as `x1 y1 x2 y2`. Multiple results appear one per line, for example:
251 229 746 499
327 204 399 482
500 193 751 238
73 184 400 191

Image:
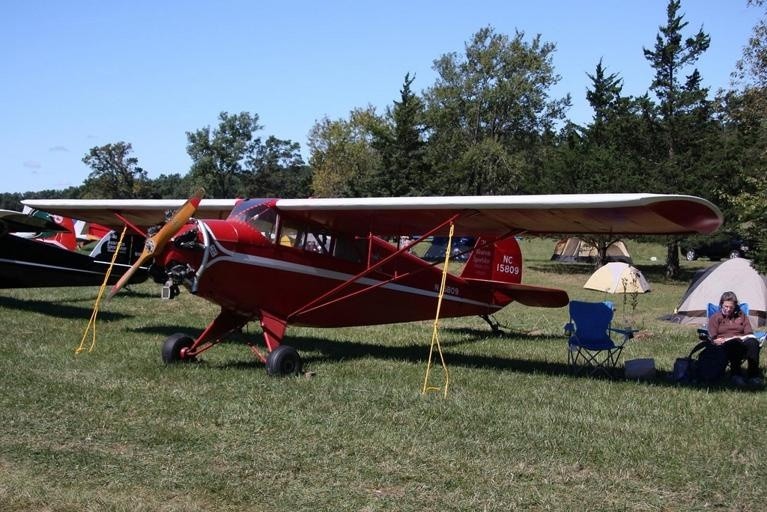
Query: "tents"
551 235 632 264
582 261 651 294
669 258 767 327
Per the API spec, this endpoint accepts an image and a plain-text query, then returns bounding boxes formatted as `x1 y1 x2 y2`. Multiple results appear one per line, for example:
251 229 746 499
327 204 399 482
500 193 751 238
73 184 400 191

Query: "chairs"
696 302 767 370
563 300 634 370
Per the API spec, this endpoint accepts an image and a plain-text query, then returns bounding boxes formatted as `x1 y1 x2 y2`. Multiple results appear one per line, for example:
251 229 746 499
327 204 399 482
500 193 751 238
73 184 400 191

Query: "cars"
681 231 764 260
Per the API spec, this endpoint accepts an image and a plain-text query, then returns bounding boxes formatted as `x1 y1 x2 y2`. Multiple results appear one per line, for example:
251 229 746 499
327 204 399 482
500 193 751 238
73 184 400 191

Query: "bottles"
570 331 577 352
624 319 634 339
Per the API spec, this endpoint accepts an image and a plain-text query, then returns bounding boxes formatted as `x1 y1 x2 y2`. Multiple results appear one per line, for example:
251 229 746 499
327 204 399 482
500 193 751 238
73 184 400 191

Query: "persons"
708 292 764 384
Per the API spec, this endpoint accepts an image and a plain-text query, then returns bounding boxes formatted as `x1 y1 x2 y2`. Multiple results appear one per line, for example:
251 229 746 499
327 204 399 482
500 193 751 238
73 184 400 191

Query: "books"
724 334 756 342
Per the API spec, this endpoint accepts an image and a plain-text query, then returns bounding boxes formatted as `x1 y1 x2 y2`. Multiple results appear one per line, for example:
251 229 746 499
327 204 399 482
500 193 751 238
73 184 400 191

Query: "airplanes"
0 208 151 294
19 188 725 379
